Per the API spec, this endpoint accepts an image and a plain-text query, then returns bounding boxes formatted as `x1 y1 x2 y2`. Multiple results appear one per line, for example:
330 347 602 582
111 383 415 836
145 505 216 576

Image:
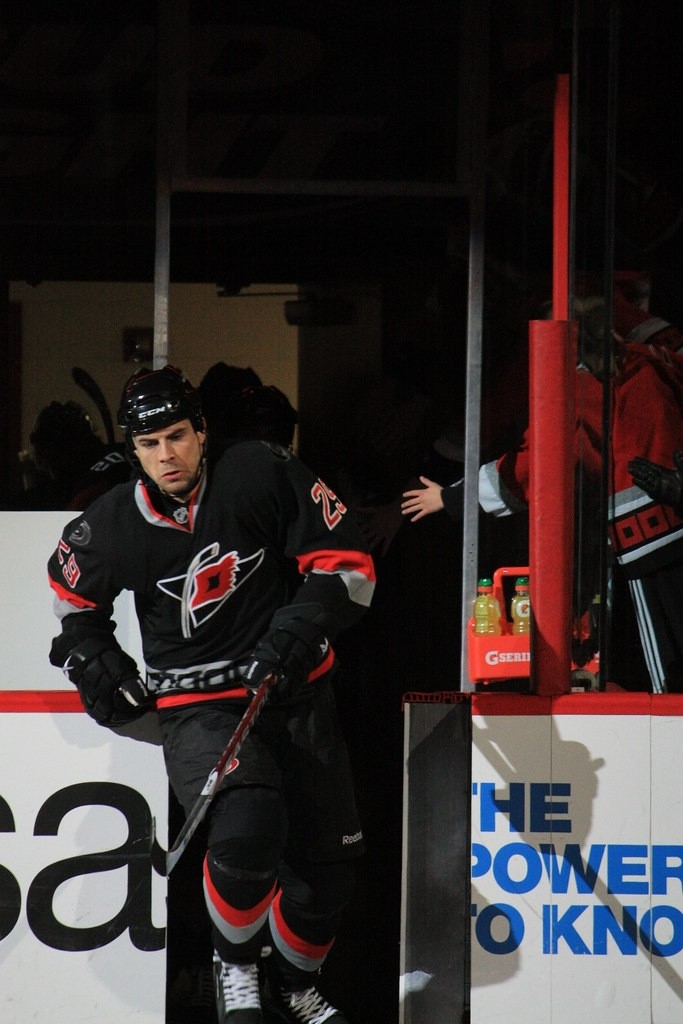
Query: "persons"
31 363 298 511
402 268 683 696
47 363 377 1024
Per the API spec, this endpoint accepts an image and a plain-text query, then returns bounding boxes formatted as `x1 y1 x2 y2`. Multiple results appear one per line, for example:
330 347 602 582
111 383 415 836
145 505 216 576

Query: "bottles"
472 579 501 635
511 577 530 634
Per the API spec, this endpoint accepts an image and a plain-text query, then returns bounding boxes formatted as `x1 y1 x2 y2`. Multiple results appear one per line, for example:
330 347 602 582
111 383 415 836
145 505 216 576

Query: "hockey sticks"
150 672 280 877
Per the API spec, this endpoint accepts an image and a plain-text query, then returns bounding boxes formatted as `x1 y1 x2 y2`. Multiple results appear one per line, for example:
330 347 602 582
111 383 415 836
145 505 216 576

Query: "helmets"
117 364 204 436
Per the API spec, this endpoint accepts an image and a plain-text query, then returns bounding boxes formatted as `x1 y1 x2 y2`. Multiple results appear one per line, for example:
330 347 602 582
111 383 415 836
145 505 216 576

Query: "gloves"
628 449 683 518
234 600 336 712
50 620 151 727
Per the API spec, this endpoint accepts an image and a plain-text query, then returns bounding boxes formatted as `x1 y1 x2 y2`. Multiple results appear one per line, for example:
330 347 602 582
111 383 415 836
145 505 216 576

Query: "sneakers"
261 950 344 1023
211 941 273 1024
